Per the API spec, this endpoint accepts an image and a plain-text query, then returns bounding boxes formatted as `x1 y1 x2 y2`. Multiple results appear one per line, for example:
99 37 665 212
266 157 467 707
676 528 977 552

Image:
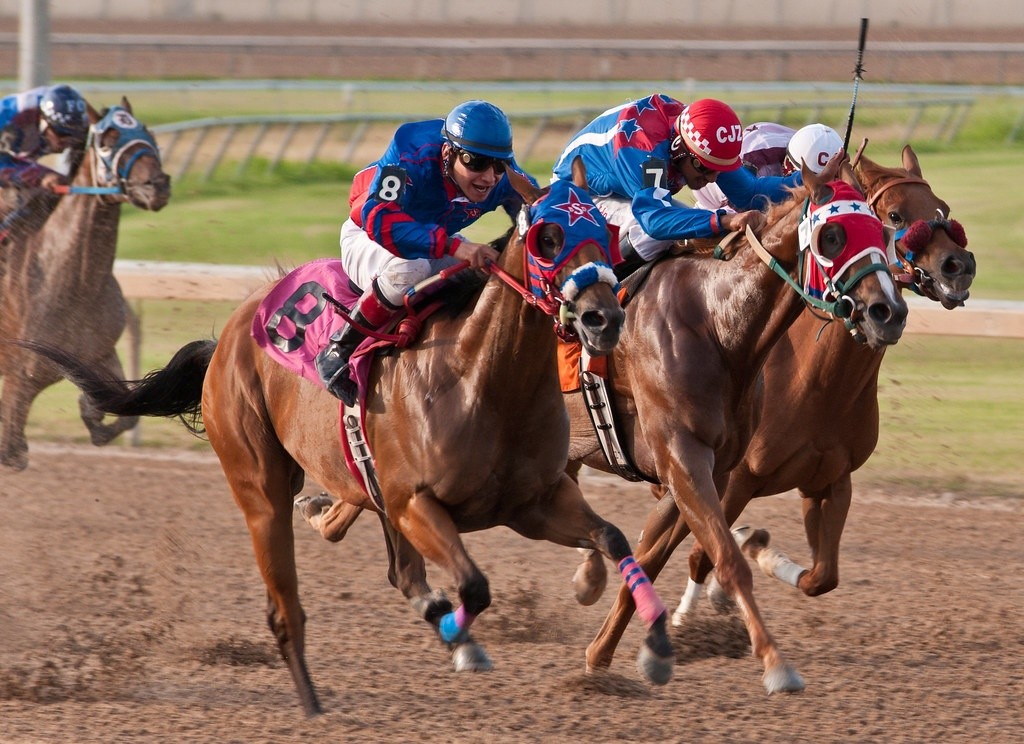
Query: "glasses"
448 139 512 174
40 110 86 140
679 137 720 177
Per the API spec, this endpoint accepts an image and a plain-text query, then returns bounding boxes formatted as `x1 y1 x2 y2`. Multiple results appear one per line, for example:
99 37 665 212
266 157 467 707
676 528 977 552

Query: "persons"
548 92 844 282
315 100 540 407
0 81 92 245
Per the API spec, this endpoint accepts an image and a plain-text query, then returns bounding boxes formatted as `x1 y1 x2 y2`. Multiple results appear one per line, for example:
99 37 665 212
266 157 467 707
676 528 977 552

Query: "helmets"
787 123 845 174
39 84 88 139
674 99 743 172
441 99 514 159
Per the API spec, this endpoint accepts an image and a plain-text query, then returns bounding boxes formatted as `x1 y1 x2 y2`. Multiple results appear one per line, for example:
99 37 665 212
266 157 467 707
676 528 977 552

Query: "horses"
0 94 172 478
6 142 978 724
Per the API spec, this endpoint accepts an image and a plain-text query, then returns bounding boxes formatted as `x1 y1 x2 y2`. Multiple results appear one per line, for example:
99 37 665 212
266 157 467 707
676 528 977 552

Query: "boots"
314 279 404 407
610 231 657 282
